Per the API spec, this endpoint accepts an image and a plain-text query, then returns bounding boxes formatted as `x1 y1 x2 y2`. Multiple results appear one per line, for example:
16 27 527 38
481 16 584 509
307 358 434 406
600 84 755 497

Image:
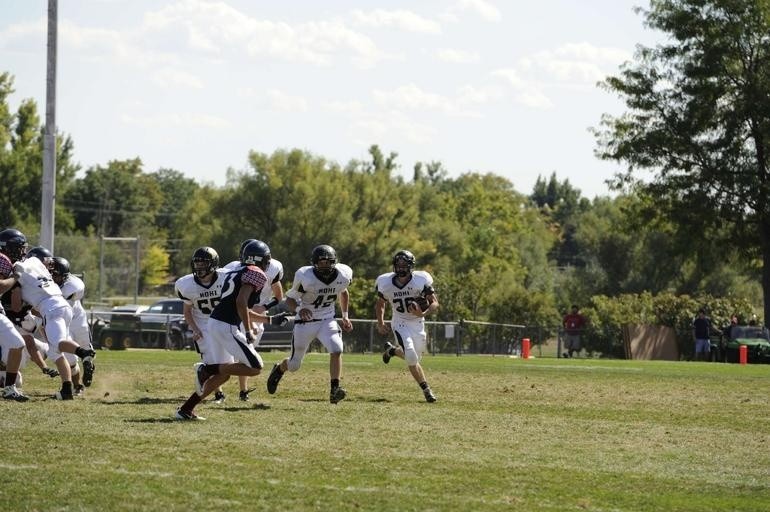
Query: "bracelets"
341 312 348 319
295 305 304 314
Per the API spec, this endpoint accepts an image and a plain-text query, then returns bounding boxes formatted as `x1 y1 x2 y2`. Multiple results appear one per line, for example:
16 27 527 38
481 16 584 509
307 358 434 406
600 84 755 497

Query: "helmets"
48 257 70 287
392 250 415 277
239 239 255 259
26 247 52 264
242 240 271 272
311 245 337 276
0 229 28 262
191 247 219 278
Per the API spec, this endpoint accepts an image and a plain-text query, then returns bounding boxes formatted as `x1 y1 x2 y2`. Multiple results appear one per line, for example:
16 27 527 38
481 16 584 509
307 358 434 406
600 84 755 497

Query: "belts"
294 318 327 324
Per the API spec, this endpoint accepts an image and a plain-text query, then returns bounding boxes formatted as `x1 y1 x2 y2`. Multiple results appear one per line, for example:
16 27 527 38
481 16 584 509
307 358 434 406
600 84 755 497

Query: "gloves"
246 331 255 344
269 312 292 328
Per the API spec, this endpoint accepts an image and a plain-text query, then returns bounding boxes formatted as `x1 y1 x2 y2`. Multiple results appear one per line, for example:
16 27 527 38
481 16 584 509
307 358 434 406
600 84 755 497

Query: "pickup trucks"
710 324 770 363
85 304 149 350
112 299 189 349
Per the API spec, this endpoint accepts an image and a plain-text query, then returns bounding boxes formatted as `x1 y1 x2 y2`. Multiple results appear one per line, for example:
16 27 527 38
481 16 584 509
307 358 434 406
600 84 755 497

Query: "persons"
375 250 437 403
723 316 739 343
0 226 97 403
266 244 354 404
173 245 228 361
211 239 284 404
175 239 293 423
745 319 761 338
562 305 586 359
692 306 715 362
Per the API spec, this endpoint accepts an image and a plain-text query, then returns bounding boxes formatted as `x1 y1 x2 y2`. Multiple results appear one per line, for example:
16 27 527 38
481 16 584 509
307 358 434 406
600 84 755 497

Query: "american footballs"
412 297 430 312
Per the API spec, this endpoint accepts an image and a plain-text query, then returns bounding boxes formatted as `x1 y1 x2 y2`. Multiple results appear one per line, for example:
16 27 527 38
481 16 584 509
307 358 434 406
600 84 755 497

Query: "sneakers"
175 408 206 421
330 387 346 404
268 363 285 394
43 367 59 377
212 391 225 404
3 385 29 402
423 387 436 402
194 363 210 397
240 387 256 401
50 389 73 400
74 385 84 396
383 342 393 364
83 350 96 387
